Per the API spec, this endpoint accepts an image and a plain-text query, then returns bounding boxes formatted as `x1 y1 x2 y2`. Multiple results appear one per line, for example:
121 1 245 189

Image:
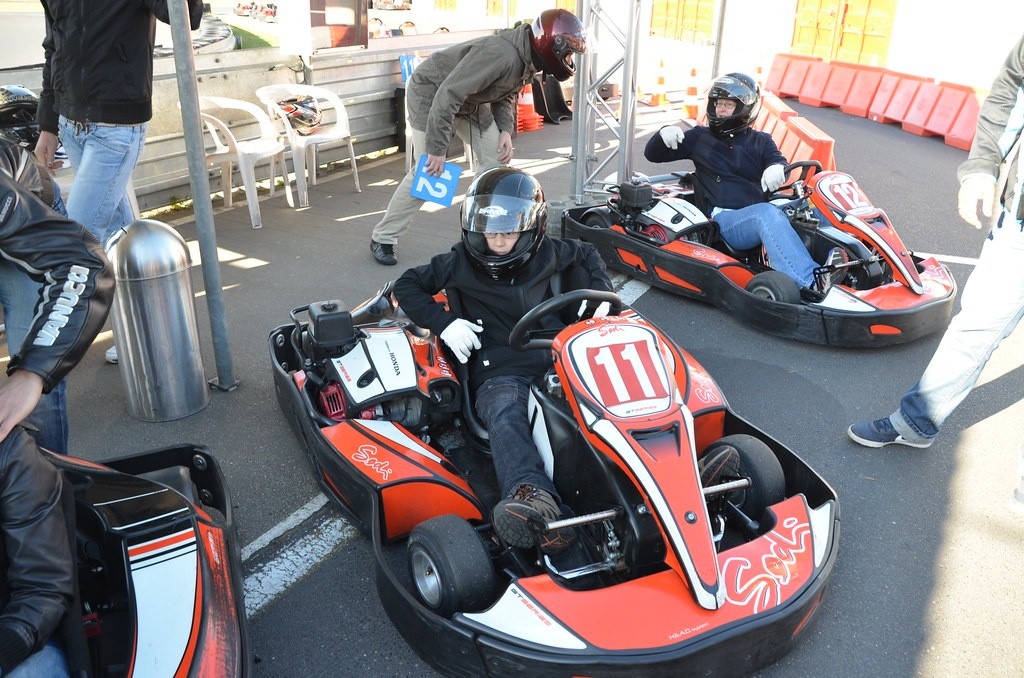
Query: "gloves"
439 318 484 364
659 126 685 150
761 164 785 192
577 299 609 318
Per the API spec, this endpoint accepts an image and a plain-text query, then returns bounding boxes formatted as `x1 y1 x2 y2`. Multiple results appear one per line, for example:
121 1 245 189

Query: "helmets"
460 166 548 281
706 73 761 137
0 85 40 152
277 95 322 136
529 8 586 81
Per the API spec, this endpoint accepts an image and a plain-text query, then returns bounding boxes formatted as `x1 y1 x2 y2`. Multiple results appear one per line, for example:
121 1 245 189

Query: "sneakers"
812 247 849 294
369 239 398 265
848 417 932 449
698 445 740 503
494 483 577 555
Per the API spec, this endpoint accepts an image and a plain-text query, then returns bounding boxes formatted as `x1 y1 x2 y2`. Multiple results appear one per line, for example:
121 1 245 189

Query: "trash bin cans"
100 218 211 423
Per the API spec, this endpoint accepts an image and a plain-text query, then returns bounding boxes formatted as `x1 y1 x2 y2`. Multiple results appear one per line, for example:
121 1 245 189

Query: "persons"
0 424 76 678
34 0 204 364
393 167 742 556
369 9 586 265
0 133 117 456
644 73 848 288
847 37 1024 448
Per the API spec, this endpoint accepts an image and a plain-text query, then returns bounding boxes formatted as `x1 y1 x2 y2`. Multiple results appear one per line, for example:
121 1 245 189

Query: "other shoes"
106 345 118 363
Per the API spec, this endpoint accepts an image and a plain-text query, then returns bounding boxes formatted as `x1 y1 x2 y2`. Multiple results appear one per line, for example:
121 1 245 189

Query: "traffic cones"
515 82 544 133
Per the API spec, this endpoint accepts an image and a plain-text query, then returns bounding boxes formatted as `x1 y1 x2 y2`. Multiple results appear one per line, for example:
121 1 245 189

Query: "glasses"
713 102 736 109
479 232 520 239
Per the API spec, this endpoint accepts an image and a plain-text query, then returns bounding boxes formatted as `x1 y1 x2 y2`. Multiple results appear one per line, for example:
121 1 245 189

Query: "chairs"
178 96 295 229
256 82 362 206
403 68 475 171
